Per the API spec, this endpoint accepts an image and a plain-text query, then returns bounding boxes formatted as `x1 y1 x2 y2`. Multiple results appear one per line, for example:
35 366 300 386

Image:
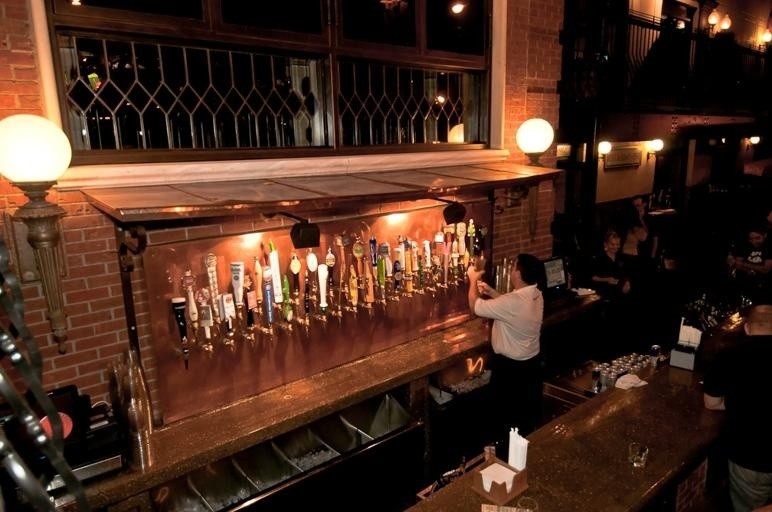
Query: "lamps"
431 197 467 225
1 112 73 355
597 141 612 162
646 139 664 161
707 8 731 34
515 116 554 243
261 211 320 249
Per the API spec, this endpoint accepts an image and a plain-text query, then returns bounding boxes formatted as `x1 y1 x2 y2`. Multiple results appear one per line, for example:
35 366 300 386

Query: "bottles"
591 352 650 394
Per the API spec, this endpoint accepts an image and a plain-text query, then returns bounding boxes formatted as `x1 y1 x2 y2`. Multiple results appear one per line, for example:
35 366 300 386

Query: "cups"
628 442 649 468
515 497 539 512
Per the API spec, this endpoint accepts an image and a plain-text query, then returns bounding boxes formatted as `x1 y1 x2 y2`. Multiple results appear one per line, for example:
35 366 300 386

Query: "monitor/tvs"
540 257 568 293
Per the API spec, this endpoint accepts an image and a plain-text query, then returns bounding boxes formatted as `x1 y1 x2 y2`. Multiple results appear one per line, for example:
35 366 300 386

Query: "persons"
467 250 549 446
700 302 772 512
583 196 771 301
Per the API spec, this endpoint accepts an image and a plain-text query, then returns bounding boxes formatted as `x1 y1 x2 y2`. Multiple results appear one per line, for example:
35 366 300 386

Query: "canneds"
591 352 650 393
